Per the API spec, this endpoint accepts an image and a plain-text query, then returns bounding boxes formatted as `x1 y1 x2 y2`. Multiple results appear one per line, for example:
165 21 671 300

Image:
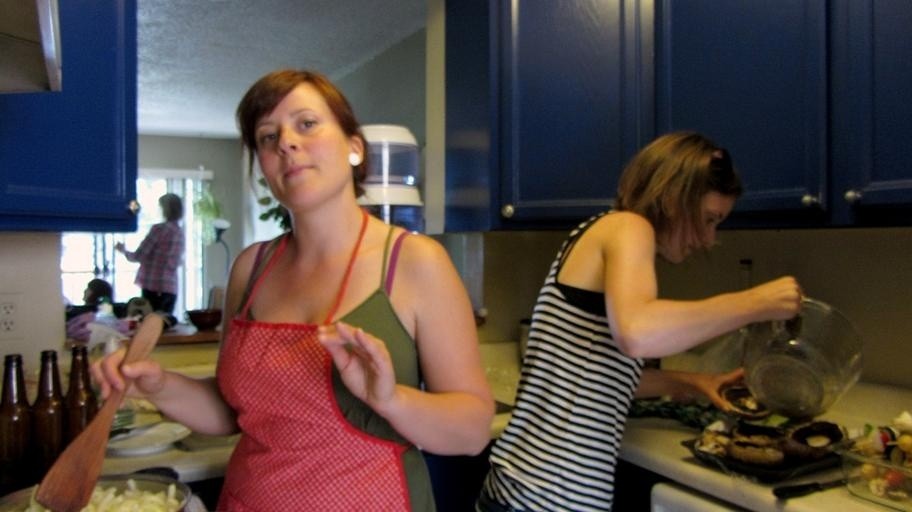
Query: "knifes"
772 476 852 502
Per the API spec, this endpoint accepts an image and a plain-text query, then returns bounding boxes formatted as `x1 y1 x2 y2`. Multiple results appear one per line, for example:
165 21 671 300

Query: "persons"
113 191 187 319
87 70 497 512
81 278 113 307
468 131 805 511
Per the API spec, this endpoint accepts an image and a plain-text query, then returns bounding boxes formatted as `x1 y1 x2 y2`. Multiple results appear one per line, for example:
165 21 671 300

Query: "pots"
1 466 194 512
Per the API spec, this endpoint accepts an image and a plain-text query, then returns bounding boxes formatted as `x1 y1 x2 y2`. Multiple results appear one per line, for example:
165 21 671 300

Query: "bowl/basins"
832 440 912 509
186 306 223 334
735 292 872 417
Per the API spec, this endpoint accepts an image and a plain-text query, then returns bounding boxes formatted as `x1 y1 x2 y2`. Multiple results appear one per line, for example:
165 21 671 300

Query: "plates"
676 433 853 482
110 424 192 460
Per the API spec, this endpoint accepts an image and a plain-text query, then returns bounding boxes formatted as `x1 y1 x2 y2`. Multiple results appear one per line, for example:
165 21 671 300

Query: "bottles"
1 342 99 496
97 296 114 328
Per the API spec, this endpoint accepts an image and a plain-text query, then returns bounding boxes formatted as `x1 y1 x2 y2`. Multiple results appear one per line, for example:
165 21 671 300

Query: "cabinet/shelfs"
447 1 645 232
1 3 137 230
649 2 911 213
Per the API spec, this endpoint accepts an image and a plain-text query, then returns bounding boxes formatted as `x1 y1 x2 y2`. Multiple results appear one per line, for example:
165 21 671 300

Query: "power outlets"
0 291 25 340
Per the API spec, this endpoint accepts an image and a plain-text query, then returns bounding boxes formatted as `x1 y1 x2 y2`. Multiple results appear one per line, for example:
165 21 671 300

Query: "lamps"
212 218 235 277
356 122 425 226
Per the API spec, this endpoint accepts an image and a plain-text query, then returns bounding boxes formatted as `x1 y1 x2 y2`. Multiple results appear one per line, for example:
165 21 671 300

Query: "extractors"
0 0 67 96
